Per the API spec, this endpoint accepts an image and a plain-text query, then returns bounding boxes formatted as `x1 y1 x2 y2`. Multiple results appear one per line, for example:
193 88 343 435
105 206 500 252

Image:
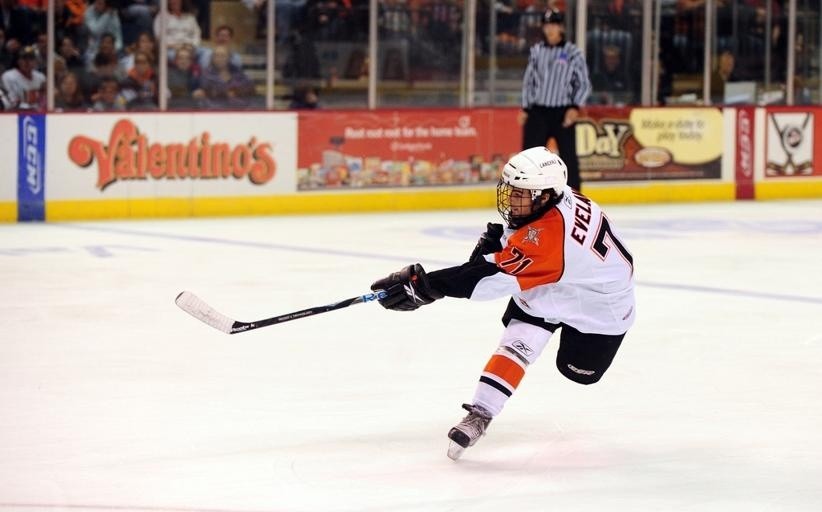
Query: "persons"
518 9 595 195
369 145 639 452
1 1 822 113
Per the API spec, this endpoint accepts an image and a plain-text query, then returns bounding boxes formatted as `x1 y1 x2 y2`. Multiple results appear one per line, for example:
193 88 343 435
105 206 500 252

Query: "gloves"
371 263 444 311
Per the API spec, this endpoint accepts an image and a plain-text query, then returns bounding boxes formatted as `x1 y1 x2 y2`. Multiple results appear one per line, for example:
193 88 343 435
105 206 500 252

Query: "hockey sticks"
176 291 389 335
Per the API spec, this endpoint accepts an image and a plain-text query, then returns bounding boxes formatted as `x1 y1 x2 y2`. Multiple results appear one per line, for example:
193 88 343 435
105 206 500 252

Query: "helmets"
542 9 564 23
496 146 567 228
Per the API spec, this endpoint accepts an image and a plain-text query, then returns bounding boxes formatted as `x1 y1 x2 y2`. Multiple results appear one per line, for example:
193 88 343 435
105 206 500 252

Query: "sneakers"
448 403 492 447
